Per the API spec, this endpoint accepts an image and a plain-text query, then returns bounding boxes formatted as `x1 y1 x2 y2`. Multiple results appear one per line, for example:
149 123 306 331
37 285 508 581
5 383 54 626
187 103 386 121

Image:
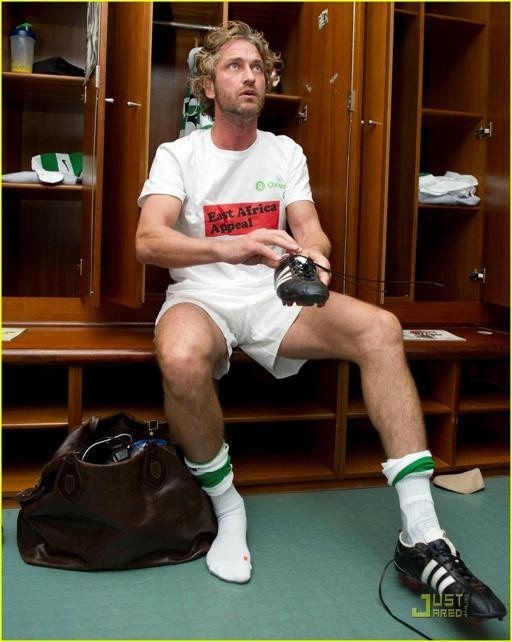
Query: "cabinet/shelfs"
2 1 510 508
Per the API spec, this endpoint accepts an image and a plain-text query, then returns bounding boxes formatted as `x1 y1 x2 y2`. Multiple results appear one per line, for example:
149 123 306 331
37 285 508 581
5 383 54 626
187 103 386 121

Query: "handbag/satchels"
14 408 219 573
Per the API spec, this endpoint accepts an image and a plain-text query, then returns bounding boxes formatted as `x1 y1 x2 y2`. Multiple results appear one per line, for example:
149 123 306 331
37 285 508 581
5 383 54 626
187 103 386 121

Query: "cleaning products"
10 23 35 74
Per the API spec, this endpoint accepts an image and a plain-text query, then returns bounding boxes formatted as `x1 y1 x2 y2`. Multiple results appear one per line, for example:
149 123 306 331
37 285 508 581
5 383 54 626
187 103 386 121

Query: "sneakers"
273 252 331 308
394 528 508 627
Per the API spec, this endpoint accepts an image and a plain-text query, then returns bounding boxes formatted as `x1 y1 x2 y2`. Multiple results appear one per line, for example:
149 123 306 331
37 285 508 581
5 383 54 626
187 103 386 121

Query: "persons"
133 18 509 627
